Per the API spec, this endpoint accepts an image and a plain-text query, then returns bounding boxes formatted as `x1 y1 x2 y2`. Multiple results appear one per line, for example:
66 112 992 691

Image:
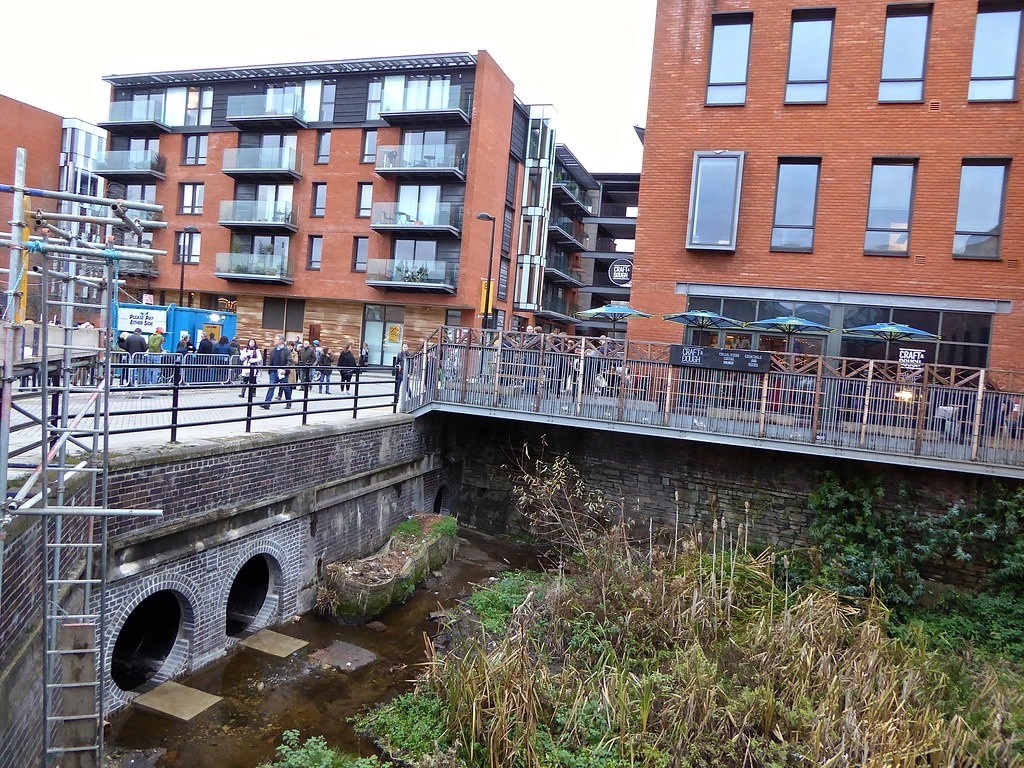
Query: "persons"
19 318 369 410
391 341 414 405
501 324 634 400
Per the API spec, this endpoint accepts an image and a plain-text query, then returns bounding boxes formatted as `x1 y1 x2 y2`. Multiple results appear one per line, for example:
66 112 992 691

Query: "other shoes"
347 391 350 394
285 402 291 409
252 393 256 397
340 391 345 394
239 393 244 398
260 404 270 409
275 396 281 400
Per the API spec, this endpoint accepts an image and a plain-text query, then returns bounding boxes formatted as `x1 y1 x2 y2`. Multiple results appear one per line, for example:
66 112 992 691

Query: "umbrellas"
576 304 654 341
747 316 837 372
844 322 941 382
663 310 747 346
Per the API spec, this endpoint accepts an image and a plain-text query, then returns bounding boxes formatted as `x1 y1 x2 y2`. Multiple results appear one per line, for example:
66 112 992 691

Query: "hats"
313 340 319 344
